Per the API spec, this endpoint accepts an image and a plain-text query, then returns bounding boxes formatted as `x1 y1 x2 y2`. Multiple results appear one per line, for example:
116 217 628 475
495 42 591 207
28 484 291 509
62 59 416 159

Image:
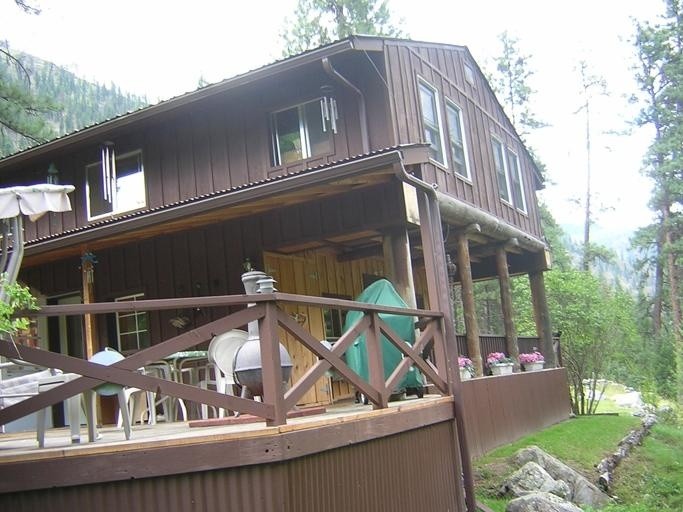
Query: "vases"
460 367 472 380
491 363 515 376
523 361 544 371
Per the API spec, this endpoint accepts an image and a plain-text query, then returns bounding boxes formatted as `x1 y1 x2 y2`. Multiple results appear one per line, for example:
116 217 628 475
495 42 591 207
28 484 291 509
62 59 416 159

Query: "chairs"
176 365 218 420
105 345 174 428
206 329 301 418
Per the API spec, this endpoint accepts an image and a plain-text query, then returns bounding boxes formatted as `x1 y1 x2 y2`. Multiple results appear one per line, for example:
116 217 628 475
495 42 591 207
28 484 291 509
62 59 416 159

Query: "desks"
165 351 212 421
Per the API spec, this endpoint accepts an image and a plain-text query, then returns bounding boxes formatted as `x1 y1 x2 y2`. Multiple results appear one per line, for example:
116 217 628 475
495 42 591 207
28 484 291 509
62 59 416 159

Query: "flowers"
519 351 545 362
458 356 475 376
486 351 516 370
